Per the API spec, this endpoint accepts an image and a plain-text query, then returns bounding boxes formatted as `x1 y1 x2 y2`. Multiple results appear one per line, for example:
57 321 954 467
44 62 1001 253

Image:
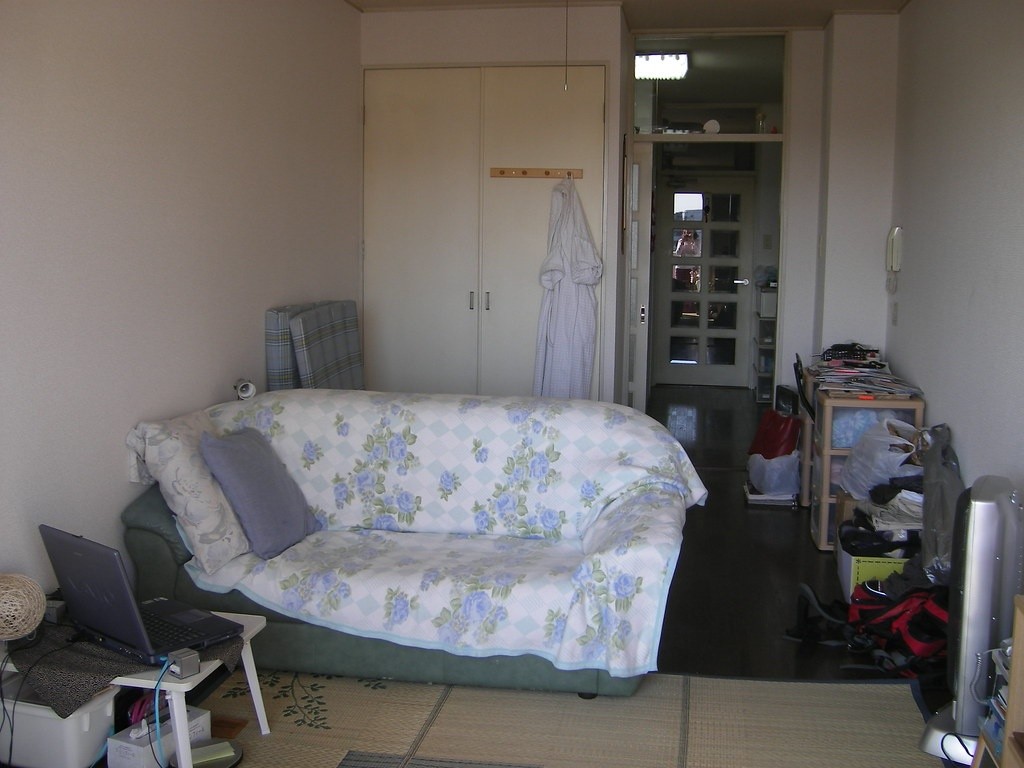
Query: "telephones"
886 226 903 272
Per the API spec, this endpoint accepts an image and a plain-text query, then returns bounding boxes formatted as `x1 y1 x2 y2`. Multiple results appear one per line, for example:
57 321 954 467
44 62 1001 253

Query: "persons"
676 229 699 284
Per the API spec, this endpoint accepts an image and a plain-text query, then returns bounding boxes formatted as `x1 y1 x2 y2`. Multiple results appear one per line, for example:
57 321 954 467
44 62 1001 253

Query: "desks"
0 609 270 768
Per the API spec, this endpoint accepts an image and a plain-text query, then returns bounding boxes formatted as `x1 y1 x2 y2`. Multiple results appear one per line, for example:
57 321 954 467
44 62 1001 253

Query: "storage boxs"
756 286 778 317
0 674 121 768
834 489 910 605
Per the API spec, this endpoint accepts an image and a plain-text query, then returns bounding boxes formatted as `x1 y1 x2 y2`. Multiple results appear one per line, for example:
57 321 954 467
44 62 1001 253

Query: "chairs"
793 352 814 420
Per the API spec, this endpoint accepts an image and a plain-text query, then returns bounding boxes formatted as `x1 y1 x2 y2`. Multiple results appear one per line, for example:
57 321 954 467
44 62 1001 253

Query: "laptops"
38 524 244 665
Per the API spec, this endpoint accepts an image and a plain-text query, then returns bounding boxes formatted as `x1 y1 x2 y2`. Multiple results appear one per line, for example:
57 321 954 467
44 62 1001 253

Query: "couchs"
119 389 709 700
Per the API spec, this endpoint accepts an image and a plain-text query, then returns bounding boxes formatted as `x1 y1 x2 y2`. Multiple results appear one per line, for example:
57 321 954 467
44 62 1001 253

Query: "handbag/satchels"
839 418 928 501
752 408 801 459
745 448 801 496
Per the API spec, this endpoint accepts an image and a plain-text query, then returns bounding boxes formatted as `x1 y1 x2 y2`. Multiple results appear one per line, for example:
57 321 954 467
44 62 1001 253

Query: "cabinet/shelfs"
970 593 1024 767
753 312 777 404
357 62 608 402
800 367 924 551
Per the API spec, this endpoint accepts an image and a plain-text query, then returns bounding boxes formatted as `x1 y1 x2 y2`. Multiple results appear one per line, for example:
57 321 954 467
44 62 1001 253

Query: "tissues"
107 699 211 768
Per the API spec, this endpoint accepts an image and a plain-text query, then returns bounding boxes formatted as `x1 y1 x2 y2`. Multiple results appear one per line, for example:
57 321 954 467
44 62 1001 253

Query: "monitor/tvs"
918 474 1019 766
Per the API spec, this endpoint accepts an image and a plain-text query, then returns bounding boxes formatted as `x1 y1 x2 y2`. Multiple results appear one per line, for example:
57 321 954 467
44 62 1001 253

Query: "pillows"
199 427 322 559
